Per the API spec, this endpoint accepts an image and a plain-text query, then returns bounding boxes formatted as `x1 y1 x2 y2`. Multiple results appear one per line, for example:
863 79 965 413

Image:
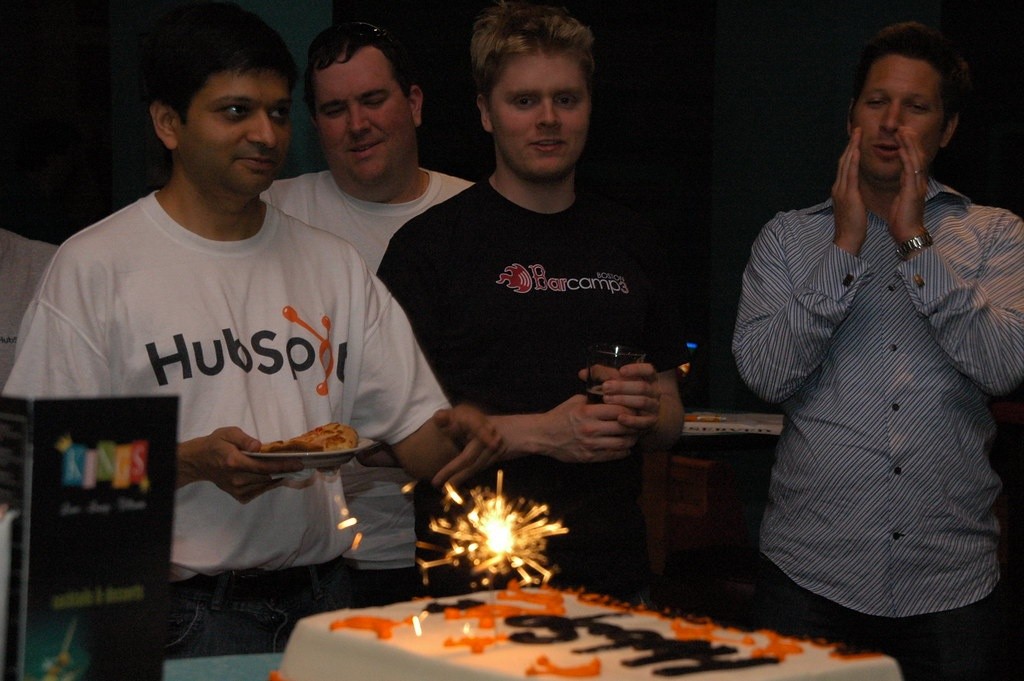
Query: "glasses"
307 22 412 80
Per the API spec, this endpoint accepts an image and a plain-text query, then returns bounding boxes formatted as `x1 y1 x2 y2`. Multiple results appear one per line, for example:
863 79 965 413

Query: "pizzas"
257 422 360 454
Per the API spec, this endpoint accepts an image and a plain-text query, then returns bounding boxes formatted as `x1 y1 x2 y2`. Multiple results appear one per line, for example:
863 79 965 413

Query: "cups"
585 342 646 409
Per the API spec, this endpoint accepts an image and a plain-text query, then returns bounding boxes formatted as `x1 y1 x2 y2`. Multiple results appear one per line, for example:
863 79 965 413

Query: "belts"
172 555 343 600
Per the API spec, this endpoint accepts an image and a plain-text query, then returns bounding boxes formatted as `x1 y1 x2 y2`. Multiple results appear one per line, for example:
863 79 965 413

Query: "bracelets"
896 232 934 258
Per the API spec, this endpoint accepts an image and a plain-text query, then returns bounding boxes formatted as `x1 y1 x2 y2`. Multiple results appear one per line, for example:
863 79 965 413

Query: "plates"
241 438 374 469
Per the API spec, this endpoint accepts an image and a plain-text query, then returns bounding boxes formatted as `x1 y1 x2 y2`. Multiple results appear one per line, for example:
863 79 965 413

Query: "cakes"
270 586 907 681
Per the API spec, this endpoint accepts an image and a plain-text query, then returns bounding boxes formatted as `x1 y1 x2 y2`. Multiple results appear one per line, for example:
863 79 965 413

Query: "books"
0 391 184 681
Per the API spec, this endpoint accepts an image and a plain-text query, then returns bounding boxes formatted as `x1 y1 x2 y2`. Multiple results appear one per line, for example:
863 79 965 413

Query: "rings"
915 169 923 175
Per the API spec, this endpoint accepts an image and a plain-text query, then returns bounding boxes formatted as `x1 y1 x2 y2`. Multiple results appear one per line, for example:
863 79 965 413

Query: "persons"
377 0 687 610
0 0 507 681
260 18 478 614
731 20 1024 681
0 226 58 398
